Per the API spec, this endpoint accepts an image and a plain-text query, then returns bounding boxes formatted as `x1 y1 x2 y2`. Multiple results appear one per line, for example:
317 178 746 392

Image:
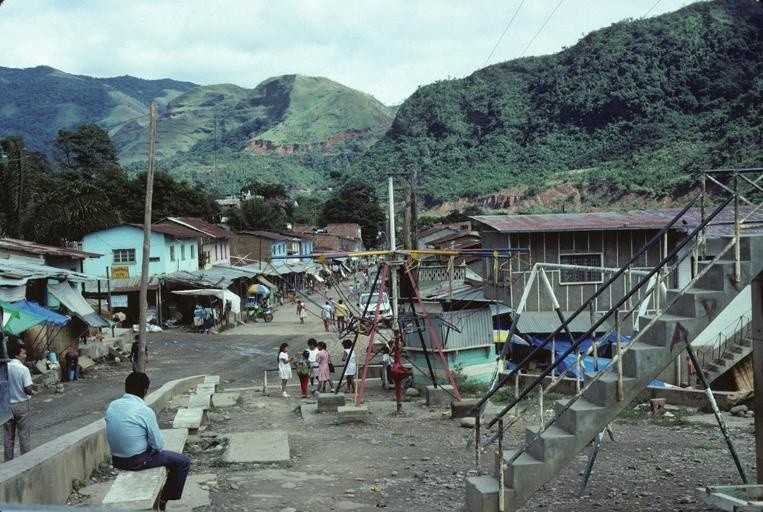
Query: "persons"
334 299 346 330
246 283 270 306
129 335 149 372
313 341 335 395
103 372 191 512
341 339 355 393
192 302 218 335
382 345 392 386
294 338 322 391
295 300 306 324
320 301 332 331
261 271 369 312
276 343 294 396
2 340 32 463
293 350 309 397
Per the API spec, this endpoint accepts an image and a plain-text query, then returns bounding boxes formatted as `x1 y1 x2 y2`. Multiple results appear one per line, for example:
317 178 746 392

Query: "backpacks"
295 358 311 378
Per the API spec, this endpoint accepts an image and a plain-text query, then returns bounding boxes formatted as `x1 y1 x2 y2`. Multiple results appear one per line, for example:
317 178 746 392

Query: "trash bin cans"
65 349 78 381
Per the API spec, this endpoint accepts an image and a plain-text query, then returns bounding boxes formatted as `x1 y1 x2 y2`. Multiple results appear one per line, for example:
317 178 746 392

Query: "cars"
369 275 384 289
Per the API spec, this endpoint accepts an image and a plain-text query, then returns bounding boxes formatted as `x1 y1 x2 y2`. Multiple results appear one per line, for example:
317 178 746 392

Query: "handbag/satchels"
329 363 336 373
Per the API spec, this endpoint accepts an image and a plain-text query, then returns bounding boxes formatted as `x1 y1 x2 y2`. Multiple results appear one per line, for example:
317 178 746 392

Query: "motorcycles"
246 304 261 321
257 306 273 323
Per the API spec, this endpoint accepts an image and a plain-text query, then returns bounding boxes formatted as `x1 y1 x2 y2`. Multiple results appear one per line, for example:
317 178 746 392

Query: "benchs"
102 373 220 511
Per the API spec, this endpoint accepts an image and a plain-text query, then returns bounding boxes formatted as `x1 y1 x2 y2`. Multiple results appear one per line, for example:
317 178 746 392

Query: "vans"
356 291 393 325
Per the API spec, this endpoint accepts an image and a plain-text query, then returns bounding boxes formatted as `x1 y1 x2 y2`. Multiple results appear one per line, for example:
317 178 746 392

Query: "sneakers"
160 497 168 510
281 388 355 398
